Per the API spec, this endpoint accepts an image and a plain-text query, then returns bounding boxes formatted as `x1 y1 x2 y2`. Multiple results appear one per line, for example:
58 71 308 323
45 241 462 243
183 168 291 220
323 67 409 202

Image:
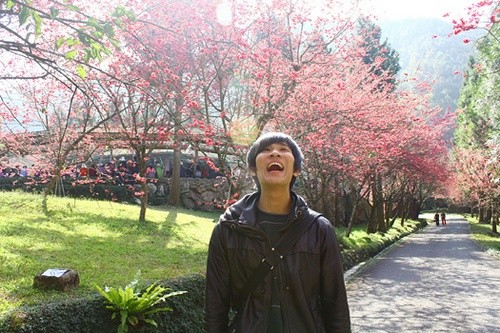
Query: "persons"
77 155 209 179
203 131 352 333
441 211 446 225
2 163 46 176
435 212 439 225
